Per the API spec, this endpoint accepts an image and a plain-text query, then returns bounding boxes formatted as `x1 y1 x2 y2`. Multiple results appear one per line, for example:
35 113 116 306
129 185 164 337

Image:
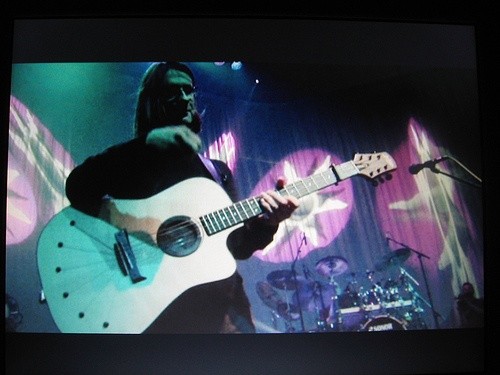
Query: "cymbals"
267 269 307 290
256 281 285 314
374 247 411 271
277 303 302 321
314 255 349 277
293 281 336 312
356 270 383 286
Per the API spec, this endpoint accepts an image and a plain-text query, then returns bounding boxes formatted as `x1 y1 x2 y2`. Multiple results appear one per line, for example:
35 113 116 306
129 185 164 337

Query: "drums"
380 286 416 308
360 289 381 312
329 291 360 314
351 312 407 332
315 305 340 328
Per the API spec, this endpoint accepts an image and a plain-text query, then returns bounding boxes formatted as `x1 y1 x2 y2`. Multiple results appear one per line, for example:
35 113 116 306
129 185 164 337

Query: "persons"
461 282 484 328
65 62 299 334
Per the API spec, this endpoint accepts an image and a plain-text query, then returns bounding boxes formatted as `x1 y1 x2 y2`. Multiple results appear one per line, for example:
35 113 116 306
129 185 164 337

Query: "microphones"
304 233 307 245
407 156 450 175
385 232 389 247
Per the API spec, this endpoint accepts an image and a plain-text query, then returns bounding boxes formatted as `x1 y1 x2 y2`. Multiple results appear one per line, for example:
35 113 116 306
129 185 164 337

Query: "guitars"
35 151 397 334
